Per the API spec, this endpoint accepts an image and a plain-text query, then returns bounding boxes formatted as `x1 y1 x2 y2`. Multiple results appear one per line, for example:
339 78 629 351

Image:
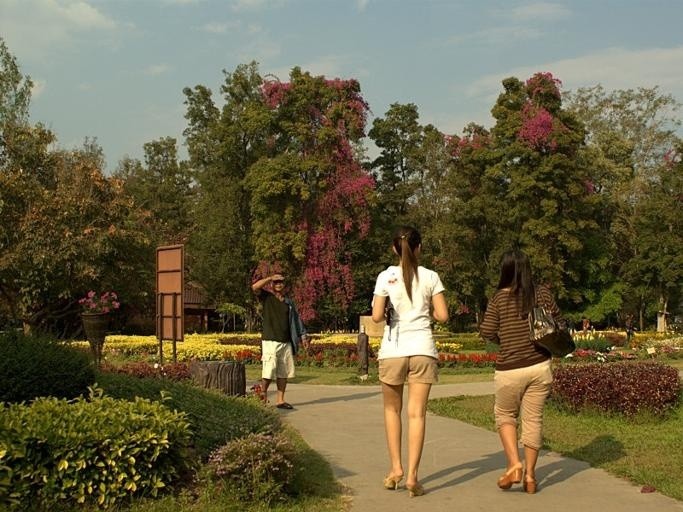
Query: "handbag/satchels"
384 296 394 325
527 281 576 357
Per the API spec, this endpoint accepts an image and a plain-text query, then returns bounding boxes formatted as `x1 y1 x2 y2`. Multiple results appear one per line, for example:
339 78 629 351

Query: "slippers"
276 402 293 409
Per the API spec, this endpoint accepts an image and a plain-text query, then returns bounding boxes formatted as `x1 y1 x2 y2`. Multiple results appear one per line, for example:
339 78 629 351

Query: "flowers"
78 288 120 314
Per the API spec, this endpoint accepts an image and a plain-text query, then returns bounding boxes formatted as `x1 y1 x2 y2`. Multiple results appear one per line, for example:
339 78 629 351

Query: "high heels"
495 466 522 489
524 477 538 493
382 473 403 490
404 480 424 498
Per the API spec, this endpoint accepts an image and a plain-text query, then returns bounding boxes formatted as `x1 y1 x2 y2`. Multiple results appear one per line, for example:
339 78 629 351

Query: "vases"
81 314 109 337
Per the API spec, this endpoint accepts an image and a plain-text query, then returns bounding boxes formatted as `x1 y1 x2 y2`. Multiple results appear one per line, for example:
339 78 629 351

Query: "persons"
251 274 308 411
478 248 568 494
370 224 451 499
